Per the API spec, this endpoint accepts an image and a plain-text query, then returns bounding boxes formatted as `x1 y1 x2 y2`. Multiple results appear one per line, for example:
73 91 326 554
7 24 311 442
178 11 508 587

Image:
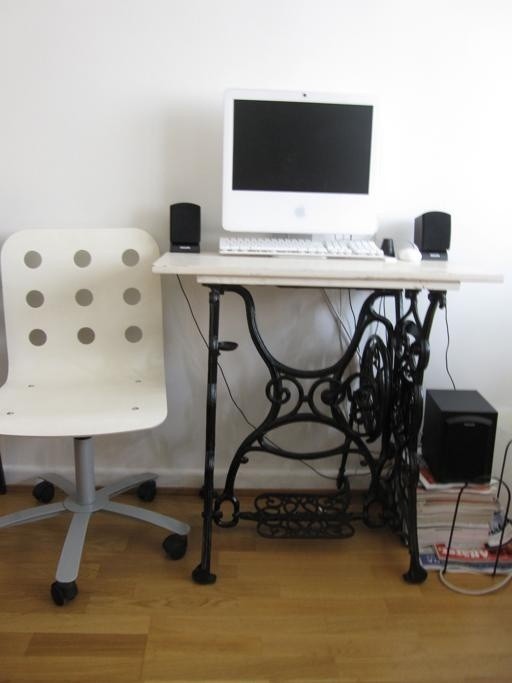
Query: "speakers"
415 212 451 260
170 203 201 253
422 390 498 484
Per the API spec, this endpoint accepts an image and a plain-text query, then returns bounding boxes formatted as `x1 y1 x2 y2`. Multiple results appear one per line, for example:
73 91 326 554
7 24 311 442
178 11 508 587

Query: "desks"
151 237 505 585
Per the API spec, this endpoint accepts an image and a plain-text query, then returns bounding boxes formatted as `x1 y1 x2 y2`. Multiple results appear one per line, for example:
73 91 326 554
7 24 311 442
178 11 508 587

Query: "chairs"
1 221 188 608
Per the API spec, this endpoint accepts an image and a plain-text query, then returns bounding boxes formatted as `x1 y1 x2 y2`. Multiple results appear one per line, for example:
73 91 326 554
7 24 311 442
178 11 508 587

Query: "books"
400 446 512 574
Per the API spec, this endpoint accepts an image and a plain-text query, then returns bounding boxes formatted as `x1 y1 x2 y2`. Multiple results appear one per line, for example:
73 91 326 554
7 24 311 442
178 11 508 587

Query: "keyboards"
219 236 385 258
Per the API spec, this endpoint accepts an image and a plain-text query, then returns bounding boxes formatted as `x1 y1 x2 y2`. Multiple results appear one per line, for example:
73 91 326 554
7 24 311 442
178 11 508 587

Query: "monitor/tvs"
222 89 383 236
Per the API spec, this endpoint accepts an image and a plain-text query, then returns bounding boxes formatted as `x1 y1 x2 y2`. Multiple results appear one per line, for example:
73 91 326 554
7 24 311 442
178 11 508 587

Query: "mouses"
398 246 422 263
